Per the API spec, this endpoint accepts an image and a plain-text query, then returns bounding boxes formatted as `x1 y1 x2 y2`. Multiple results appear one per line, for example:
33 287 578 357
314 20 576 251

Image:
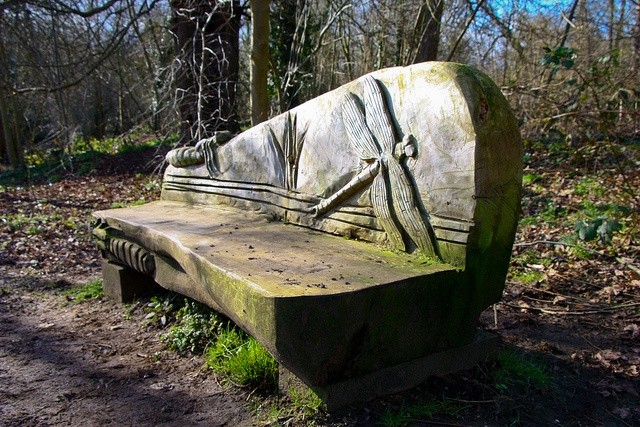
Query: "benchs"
90 62 523 412
